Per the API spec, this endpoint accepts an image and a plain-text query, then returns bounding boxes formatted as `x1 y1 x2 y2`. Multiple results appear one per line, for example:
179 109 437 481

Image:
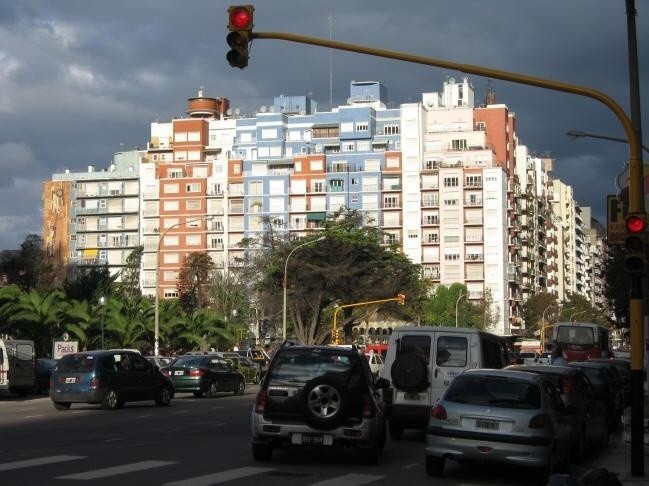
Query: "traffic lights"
623 210 648 280
225 3 253 68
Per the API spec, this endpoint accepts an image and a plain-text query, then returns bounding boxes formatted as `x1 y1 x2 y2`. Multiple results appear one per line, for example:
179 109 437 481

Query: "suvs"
247 346 387 466
48 350 175 408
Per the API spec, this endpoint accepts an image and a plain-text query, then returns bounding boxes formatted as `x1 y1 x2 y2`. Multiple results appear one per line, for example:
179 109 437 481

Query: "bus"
549 321 611 363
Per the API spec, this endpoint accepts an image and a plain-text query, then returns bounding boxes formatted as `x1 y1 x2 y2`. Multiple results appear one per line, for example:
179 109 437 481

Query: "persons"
258 344 270 382
549 346 569 365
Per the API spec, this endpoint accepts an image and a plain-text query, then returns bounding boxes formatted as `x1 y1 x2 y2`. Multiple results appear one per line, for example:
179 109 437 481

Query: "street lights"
454 292 469 327
98 296 107 349
539 303 604 346
280 235 328 346
565 127 649 159
232 309 240 353
154 215 214 358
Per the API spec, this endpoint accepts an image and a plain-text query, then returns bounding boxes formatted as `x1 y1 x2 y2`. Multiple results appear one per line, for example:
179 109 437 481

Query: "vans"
380 326 521 437
365 344 388 358
0 338 16 398
1 339 35 393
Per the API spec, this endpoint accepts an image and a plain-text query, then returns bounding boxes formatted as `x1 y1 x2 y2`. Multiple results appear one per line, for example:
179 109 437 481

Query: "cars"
36 358 58 394
361 350 385 376
537 352 551 365
425 370 583 484
518 352 540 364
145 347 271 398
503 363 609 469
567 352 634 429
